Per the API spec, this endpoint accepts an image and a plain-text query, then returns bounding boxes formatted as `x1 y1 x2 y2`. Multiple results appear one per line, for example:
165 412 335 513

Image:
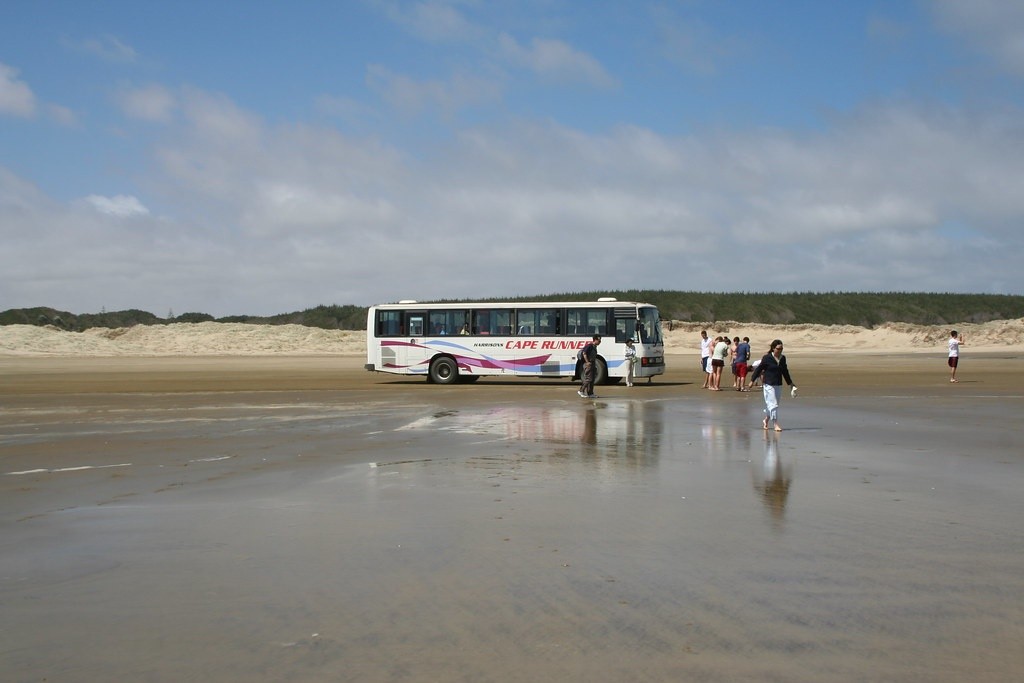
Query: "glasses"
776 348 783 350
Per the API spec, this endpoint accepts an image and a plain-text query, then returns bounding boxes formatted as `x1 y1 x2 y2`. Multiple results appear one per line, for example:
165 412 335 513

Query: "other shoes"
791 386 797 398
630 383 633 386
627 384 631 387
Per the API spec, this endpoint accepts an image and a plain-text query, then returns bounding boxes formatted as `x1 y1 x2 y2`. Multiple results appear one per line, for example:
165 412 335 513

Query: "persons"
748 339 797 431
948 330 964 383
440 324 448 334
624 339 636 387
460 323 474 335
578 334 601 398
700 330 763 392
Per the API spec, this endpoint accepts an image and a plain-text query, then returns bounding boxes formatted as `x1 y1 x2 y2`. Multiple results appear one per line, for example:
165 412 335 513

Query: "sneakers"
587 394 599 398
578 391 587 397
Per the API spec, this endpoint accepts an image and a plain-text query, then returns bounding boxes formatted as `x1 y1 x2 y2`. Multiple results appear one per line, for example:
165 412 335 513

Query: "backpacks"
577 344 593 361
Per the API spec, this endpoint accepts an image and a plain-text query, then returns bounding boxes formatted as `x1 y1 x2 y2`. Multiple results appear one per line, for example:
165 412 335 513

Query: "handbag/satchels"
631 354 638 363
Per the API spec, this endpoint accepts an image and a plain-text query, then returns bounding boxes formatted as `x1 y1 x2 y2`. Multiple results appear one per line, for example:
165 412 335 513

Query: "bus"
365 297 674 386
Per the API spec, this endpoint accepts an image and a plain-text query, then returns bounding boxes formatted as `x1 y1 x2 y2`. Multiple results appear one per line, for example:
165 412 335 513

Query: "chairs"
576 326 595 333
568 325 575 333
540 326 552 333
497 326 512 334
519 326 531 334
598 325 606 333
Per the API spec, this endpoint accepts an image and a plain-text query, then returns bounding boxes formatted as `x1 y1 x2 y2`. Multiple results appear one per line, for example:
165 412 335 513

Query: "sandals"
737 388 741 391
740 388 750 392
